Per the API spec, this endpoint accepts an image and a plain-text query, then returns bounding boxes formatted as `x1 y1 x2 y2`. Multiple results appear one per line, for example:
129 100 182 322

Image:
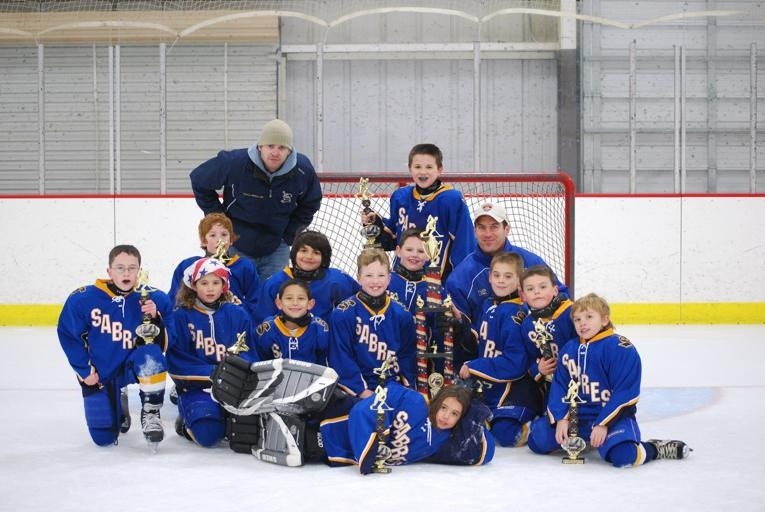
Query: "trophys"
213 240 229 263
132 268 161 344
415 214 452 313
370 384 392 474
528 317 555 384
354 175 383 248
232 330 250 357
561 378 588 465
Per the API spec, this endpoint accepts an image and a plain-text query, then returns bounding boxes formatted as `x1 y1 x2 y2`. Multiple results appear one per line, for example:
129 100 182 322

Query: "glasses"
111 263 139 272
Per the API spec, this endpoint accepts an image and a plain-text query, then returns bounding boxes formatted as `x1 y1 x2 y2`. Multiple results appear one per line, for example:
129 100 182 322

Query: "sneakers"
644 438 690 459
140 409 164 442
118 386 131 434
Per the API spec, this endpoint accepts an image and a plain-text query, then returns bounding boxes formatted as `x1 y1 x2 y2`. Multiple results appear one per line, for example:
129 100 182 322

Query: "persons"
352 226 451 320
311 381 495 475
190 119 323 275
360 142 476 280
328 247 417 400
239 278 329 366
56 244 173 446
526 293 688 467
164 256 259 448
520 263 578 456
444 201 571 344
167 212 261 304
252 230 361 324
442 252 548 447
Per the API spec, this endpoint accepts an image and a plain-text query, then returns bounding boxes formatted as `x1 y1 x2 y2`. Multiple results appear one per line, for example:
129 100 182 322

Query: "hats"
474 202 510 227
256 118 295 152
